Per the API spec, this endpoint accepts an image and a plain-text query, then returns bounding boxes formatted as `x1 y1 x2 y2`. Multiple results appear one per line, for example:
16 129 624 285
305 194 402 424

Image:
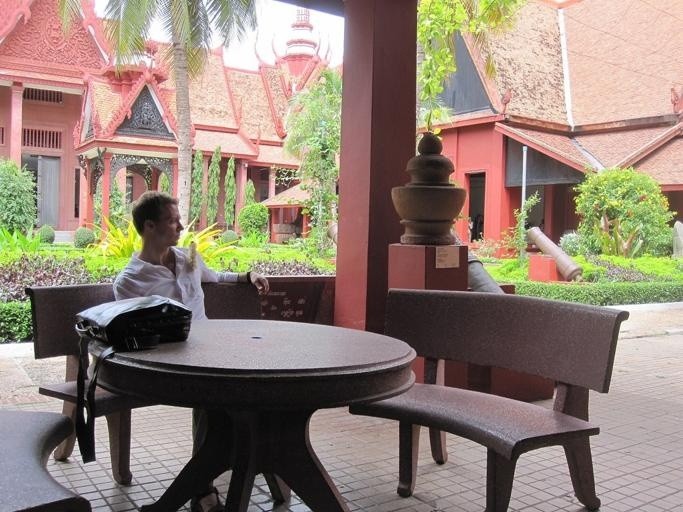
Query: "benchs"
25 281 296 505
1 407 92 512
350 289 630 512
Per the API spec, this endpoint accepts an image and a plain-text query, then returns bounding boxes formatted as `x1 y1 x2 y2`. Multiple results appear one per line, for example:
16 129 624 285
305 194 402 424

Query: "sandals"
189 487 226 512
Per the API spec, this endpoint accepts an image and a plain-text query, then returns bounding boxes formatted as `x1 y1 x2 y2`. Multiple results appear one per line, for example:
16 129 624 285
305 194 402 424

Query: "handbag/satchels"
73 295 193 464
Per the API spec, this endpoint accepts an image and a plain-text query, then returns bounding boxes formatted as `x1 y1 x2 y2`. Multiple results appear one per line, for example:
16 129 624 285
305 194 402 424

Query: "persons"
111 189 270 512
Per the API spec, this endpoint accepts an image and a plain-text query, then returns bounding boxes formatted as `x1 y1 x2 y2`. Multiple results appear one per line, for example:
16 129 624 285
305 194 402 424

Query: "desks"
89 318 416 512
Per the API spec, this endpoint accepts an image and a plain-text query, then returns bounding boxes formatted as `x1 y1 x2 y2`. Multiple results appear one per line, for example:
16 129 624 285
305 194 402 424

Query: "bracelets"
245 271 251 283
